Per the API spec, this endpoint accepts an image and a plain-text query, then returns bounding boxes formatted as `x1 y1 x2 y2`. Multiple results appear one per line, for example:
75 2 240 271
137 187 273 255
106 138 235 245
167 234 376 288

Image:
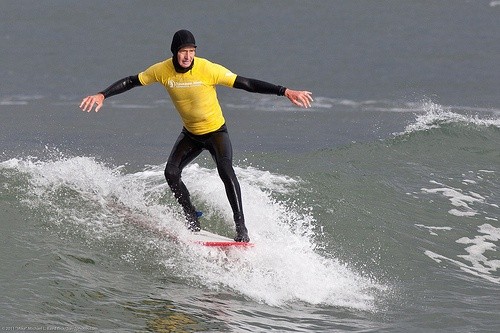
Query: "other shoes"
184 210 201 232
234 225 250 243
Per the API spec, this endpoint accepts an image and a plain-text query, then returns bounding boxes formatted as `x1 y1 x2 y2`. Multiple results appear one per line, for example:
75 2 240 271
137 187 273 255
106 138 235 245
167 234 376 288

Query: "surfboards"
119 204 254 250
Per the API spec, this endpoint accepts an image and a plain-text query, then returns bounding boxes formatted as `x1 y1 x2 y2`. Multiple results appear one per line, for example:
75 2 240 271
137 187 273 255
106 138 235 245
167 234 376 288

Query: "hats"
171 29 197 56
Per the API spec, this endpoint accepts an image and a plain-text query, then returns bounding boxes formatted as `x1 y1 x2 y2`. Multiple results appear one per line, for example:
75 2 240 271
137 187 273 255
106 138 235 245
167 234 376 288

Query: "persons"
79 29 313 243
